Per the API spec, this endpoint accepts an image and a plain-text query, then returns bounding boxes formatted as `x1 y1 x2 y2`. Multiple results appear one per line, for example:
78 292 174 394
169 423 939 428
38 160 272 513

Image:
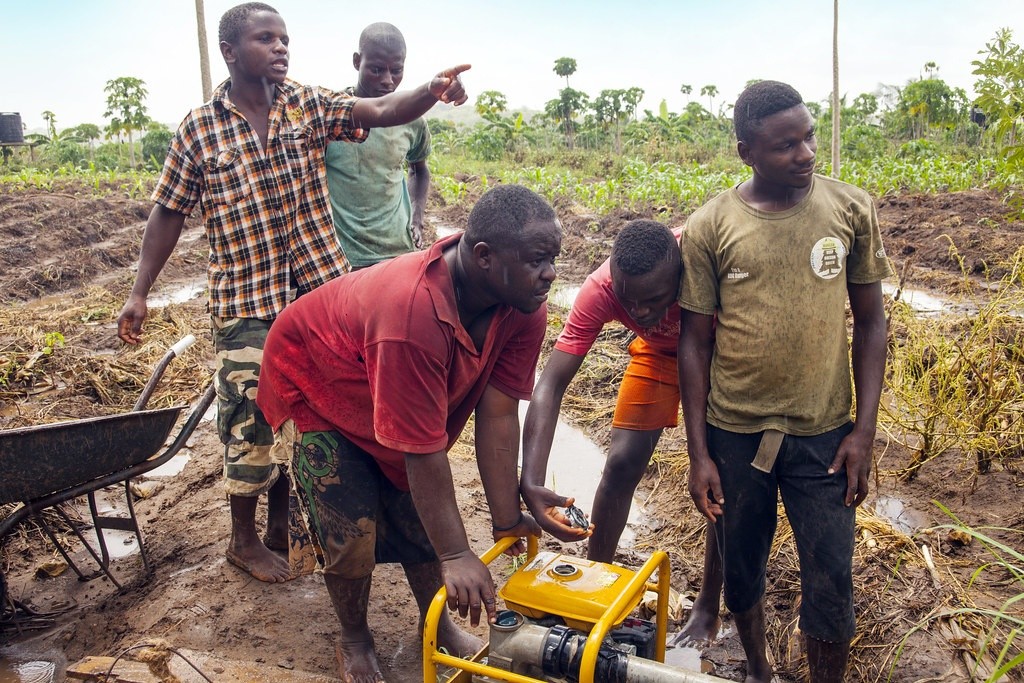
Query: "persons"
255 184 562 683
520 219 725 651
678 80 889 683
117 2 472 581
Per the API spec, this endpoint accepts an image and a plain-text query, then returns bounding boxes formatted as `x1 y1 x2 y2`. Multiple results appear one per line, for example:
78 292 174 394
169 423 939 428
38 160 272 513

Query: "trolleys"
0 334 217 615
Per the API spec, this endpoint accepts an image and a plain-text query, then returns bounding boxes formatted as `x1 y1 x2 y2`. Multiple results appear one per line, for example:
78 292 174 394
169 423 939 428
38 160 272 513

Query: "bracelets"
493 512 524 531
